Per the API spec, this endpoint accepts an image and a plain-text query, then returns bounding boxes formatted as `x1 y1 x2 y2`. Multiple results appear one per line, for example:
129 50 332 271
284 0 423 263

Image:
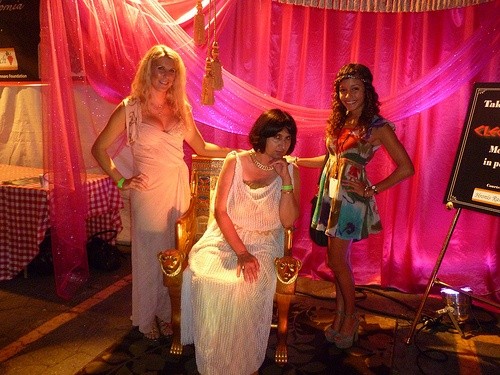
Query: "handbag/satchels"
310 196 328 246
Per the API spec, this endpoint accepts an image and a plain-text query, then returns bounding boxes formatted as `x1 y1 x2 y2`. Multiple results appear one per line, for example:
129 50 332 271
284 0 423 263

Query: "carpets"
72 277 500 375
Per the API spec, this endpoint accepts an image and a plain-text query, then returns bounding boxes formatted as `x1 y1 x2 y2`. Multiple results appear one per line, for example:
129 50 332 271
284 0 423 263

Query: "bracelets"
117 177 125 187
281 184 293 190
295 156 298 166
372 185 378 194
365 185 369 195
281 189 293 194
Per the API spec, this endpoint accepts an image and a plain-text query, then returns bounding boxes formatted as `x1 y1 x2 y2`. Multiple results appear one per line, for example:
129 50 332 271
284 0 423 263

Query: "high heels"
334 315 359 347
325 310 345 342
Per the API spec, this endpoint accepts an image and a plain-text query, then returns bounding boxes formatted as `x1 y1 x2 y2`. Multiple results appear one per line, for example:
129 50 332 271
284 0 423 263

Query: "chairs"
156 155 302 368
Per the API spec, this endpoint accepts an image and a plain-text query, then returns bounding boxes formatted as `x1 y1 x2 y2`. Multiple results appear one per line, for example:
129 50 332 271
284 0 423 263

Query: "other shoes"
143 327 160 342
158 319 174 340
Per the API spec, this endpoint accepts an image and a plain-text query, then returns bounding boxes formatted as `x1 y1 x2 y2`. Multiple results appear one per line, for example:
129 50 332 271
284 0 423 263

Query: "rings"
242 266 248 270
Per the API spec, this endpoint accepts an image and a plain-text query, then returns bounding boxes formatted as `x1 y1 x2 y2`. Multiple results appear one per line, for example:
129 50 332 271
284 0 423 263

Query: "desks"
0 163 126 282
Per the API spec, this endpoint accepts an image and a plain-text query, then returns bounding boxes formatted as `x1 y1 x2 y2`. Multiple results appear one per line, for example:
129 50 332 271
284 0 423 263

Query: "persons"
282 63 415 347
91 44 249 342
188 108 297 375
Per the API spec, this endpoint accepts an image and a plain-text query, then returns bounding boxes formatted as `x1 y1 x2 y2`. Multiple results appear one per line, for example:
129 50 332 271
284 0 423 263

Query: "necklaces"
335 120 362 164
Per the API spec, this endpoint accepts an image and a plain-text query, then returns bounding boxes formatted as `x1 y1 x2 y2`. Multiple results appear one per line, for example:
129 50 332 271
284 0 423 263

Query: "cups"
39 173 48 187
